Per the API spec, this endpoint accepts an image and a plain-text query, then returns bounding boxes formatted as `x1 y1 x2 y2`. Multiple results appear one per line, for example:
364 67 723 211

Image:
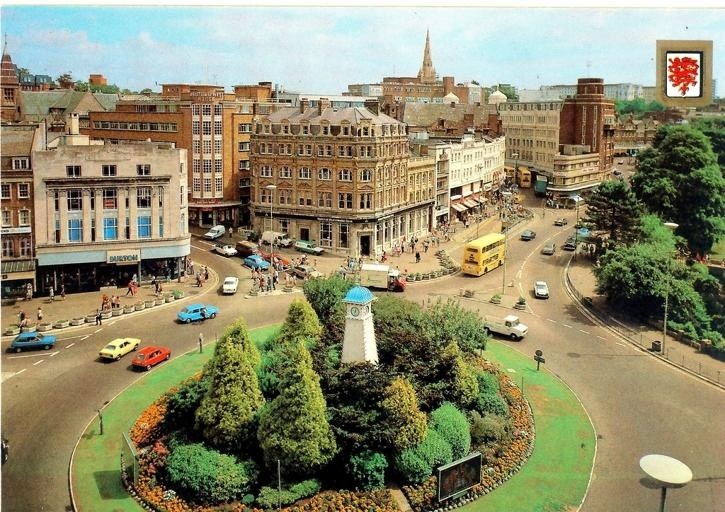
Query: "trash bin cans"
584 297 592 309
652 341 661 351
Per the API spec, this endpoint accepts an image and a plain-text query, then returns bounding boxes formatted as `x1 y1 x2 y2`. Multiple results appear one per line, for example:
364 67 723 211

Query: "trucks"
360 263 407 292
483 314 529 339
262 229 292 249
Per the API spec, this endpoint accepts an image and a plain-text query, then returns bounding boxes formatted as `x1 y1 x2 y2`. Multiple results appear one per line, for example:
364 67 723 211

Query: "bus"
504 165 548 196
461 232 508 277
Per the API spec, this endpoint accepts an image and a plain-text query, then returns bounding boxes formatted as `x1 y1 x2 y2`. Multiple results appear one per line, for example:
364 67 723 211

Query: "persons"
229 226 233 238
339 254 366 280
580 243 595 257
250 253 318 292
15 258 210 334
376 175 526 263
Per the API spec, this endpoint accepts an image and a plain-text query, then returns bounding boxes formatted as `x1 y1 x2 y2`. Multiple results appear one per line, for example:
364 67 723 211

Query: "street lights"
640 454 693 512
662 222 679 354
568 195 580 260
266 184 276 268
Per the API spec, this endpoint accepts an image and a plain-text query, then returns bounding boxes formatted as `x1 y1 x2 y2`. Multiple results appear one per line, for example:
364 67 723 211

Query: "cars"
222 276 239 295
521 229 536 241
98 337 172 371
212 240 324 279
554 218 568 226
11 330 57 353
534 281 550 298
176 303 220 324
543 227 596 256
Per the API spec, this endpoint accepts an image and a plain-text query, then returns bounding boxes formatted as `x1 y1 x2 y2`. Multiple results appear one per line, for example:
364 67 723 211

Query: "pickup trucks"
203 225 225 241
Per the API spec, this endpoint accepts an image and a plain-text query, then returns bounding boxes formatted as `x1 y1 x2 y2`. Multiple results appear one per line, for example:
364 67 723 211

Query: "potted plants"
4 288 184 336
406 249 458 282
490 292 526 310
